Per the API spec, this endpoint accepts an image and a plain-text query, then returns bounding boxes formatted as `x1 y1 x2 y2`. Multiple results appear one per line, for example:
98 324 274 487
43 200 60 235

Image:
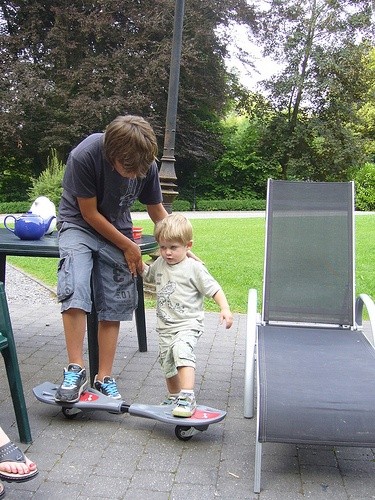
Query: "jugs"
4 211 56 240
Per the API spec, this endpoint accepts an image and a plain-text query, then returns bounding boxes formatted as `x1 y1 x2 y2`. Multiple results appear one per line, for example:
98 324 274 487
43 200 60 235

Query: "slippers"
0 441 39 483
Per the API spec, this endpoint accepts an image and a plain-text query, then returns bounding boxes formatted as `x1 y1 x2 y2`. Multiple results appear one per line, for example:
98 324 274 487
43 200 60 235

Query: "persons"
0 426 40 500
137 211 233 418
54 114 207 415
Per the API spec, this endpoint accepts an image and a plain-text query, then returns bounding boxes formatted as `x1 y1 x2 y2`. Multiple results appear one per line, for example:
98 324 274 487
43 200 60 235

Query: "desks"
0 228 167 388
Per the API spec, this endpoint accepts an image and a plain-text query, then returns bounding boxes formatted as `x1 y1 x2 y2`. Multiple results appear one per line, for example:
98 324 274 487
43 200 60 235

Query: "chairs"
243 177 375 493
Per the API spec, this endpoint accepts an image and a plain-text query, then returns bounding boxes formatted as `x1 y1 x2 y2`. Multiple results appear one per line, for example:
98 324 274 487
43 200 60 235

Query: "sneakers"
159 394 178 405
93 375 121 400
173 392 196 417
55 363 88 402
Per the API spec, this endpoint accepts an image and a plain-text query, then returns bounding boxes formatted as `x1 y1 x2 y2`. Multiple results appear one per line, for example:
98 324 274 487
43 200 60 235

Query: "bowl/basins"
132 227 143 239
